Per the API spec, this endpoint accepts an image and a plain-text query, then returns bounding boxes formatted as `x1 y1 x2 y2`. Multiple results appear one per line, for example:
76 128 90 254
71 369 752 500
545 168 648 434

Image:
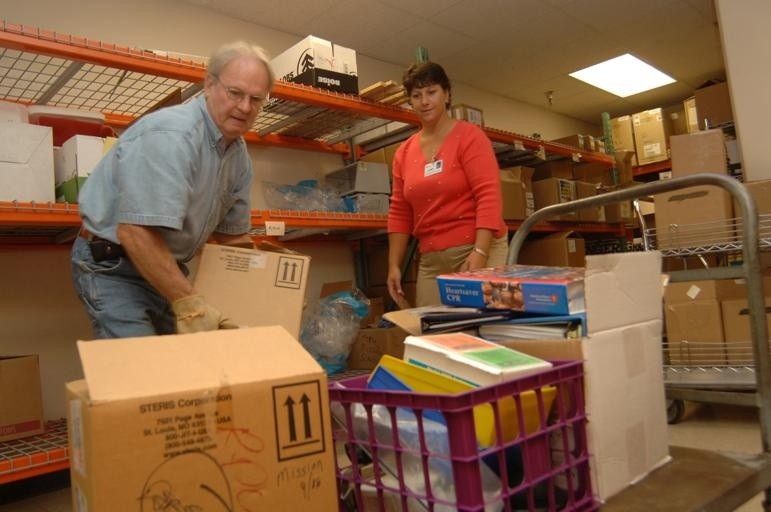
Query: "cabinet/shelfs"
2 415 68 484
250 210 388 247
643 216 771 423
3 200 80 253
1 21 208 134
244 78 423 166
326 370 373 392
503 219 627 236
481 125 617 176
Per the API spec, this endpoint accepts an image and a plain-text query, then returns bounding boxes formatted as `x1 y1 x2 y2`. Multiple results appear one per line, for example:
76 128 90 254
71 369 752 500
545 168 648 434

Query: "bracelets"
473 247 488 258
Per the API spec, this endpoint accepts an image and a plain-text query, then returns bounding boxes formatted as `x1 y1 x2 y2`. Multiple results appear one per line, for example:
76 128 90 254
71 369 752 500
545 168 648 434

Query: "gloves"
169 291 240 335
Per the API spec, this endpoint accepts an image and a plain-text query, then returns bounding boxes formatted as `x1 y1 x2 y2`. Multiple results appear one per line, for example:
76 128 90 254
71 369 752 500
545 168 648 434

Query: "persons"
69 40 274 338
385 63 513 308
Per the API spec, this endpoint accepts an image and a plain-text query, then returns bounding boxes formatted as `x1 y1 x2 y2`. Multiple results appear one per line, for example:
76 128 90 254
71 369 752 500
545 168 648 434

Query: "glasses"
215 75 266 109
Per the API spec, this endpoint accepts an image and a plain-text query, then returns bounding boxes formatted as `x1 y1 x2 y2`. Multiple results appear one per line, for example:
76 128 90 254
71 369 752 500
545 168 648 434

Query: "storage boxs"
500 166 632 227
382 245 678 504
65 322 344 512
653 84 771 369
2 120 119 202
193 244 310 347
319 250 671 484
326 143 401 210
261 34 358 119
3 352 43 441
609 107 668 171
448 104 485 128
553 133 605 155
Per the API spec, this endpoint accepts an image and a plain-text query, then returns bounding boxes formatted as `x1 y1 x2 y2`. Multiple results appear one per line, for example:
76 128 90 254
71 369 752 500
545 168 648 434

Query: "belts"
78 226 123 257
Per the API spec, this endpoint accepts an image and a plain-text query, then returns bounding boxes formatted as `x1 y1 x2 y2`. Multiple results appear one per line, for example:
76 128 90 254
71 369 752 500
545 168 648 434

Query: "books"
380 263 587 388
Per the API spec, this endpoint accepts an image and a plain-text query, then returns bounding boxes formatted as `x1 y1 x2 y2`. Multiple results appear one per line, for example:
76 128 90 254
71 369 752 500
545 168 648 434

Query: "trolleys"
633 199 769 424
508 172 771 512
